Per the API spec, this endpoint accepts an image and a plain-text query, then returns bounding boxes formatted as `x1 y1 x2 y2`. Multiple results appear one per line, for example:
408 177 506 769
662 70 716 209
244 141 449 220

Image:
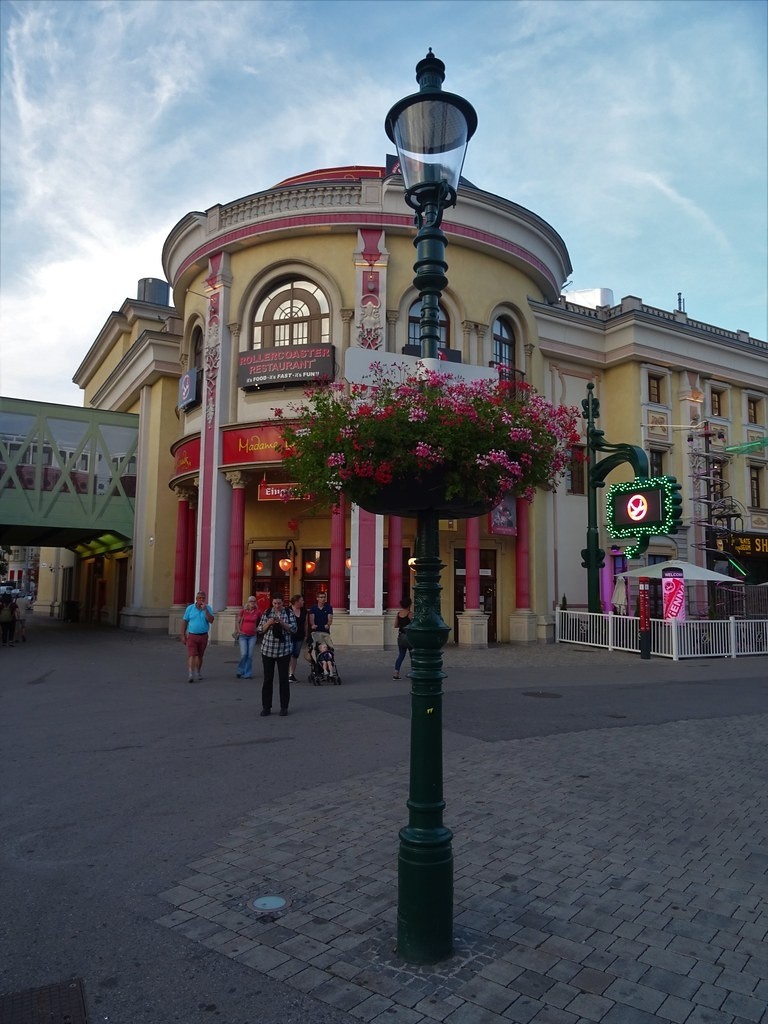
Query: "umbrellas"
610 575 628 615
610 559 745 585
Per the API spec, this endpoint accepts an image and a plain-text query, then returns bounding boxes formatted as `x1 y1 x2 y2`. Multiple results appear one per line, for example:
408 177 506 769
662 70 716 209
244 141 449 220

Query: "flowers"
262 364 591 534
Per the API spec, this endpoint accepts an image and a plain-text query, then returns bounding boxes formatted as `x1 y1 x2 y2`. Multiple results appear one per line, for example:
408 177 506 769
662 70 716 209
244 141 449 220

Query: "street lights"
383 45 481 967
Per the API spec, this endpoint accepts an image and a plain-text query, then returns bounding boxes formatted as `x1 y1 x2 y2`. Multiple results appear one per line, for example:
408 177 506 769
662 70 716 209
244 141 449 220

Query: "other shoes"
280 708 287 716
15 640 20 643
236 673 241 678
22 636 26 642
195 675 203 680
393 676 402 680
324 672 329 675
329 674 333 677
9 641 15 647
188 676 193 682
261 708 270 716
245 677 255 680
2 641 6 646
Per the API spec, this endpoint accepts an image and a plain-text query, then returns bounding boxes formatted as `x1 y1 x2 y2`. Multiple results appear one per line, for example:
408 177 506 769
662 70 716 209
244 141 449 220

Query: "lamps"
279 540 297 575
611 545 621 552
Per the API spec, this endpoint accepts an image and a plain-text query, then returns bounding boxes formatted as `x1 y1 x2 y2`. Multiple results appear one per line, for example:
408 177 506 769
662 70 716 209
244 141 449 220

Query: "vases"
349 466 505 519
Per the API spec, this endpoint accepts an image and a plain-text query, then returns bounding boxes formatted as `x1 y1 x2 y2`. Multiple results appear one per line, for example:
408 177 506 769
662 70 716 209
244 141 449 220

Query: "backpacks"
0 603 15 623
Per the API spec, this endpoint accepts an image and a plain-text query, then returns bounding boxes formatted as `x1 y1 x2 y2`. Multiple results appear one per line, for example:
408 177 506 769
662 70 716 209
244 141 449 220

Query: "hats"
18 592 25 597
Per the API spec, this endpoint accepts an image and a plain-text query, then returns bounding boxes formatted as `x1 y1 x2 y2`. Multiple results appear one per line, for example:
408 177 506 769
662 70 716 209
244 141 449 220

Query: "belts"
189 632 207 635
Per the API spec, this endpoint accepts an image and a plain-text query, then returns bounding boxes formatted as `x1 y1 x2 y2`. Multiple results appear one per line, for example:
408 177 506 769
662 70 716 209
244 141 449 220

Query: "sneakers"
289 674 298 682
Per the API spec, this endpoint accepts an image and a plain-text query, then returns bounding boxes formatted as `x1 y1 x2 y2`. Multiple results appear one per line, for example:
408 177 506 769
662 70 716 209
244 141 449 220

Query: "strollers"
308 627 342 686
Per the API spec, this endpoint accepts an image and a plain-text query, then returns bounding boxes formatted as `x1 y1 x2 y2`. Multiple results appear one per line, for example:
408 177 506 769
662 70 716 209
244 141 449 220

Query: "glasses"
250 601 256 602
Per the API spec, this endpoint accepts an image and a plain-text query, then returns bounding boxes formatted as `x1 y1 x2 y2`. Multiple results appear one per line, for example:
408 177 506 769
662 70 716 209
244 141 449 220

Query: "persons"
256 592 298 717
181 592 215 683
285 589 334 683
392 596 414 681
236 596 262 680
0 589 35 648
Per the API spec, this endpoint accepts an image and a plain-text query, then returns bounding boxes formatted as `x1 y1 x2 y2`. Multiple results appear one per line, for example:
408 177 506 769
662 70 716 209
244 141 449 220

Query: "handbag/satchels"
232 631 239 640
